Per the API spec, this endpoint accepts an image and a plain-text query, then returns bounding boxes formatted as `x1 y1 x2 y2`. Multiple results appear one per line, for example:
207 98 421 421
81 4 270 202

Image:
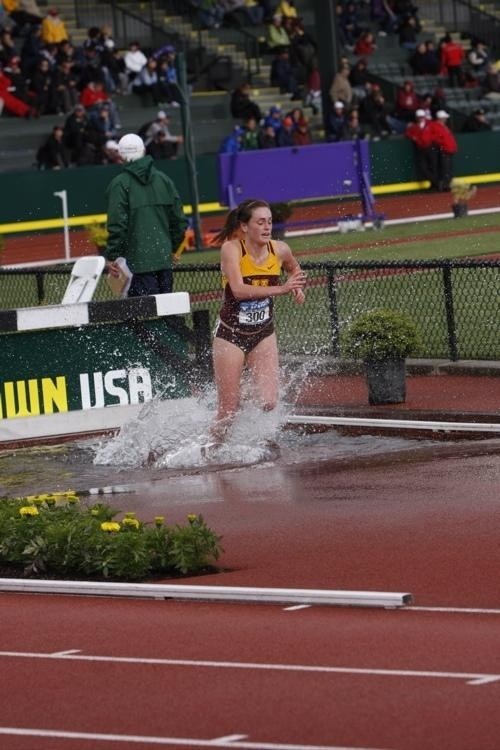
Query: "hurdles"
217 138 382 230
1 291 211 445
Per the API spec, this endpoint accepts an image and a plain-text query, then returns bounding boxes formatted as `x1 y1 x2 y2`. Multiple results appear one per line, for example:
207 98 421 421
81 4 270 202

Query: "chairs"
60 255 106 305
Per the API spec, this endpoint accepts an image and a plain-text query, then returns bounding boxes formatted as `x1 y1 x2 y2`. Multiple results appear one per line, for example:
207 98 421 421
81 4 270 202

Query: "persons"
102 133 190 294
211 199 307 452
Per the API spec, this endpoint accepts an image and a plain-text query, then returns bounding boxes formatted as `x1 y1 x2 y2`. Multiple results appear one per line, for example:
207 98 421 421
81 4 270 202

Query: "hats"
117 132 145 163
436 110 453 119
416 109 426 117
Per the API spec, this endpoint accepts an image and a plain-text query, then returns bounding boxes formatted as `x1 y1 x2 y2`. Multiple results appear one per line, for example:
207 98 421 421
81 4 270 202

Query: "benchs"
2 94 249 169
345 18 500 132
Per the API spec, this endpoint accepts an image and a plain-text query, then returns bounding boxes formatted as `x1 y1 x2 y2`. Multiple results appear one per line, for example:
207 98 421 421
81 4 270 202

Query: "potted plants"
340 304 423 406
